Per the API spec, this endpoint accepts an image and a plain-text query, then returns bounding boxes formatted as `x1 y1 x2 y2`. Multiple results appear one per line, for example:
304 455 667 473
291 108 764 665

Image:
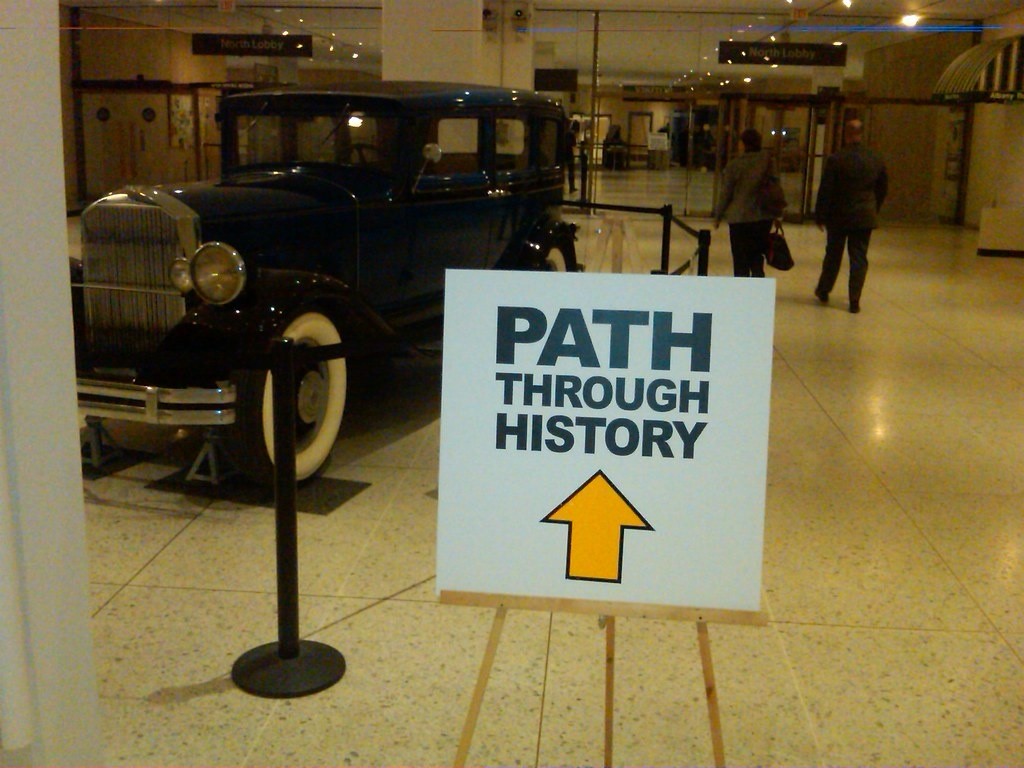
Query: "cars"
69 79 584 493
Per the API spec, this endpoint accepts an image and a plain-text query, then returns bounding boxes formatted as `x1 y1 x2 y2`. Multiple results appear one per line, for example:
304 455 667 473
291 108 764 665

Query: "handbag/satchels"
764 225 795 271
756 156 788 218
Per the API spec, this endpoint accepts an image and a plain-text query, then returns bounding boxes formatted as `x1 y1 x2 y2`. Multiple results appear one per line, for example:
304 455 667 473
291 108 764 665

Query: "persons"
713 128 784 278
814 120 889 313
678 124 714 168
605 126 626 169
658 123 669 138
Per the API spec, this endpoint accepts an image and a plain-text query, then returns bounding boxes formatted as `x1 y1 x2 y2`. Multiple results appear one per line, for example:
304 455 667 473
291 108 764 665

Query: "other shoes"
848 305 861 314
815 287 830 303
